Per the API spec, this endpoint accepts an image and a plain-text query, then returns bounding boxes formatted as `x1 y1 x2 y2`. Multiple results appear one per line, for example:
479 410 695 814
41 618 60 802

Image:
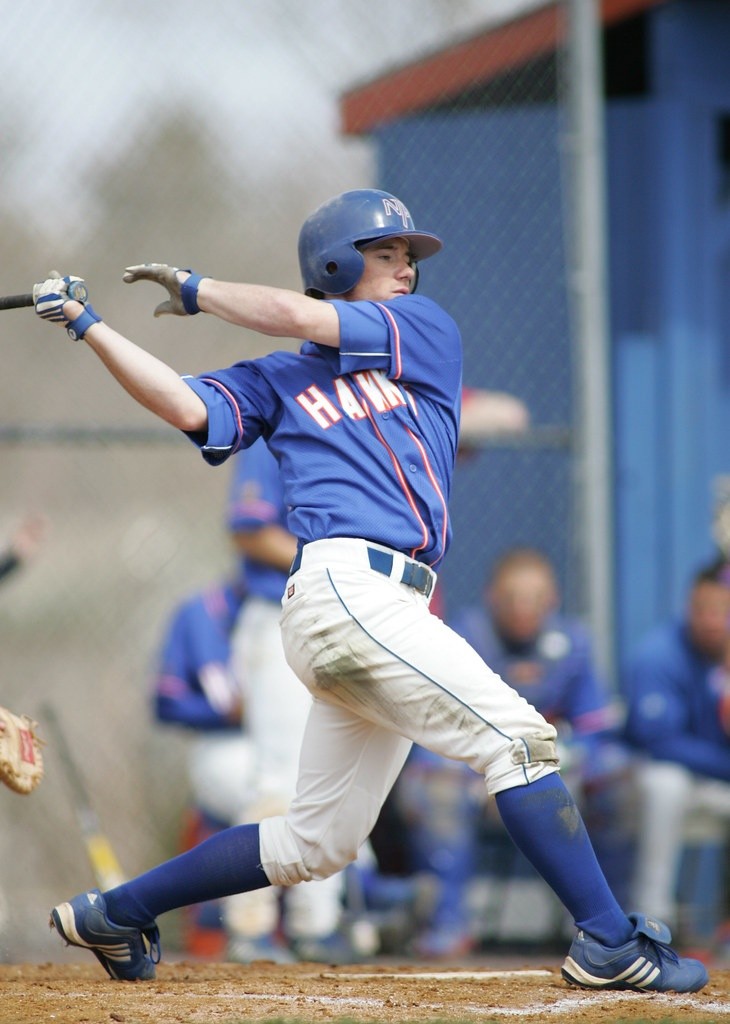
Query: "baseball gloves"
0 707 48 796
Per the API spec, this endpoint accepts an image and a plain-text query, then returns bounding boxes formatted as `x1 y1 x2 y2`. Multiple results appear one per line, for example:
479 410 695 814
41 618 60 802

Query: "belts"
290 544 433 599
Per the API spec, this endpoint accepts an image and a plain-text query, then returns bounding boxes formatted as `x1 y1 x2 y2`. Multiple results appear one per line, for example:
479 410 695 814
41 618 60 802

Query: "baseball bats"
0 281 88 310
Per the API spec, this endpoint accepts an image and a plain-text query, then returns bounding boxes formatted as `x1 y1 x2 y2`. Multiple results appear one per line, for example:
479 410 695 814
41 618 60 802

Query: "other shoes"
229 938 302 967
288 936 367 965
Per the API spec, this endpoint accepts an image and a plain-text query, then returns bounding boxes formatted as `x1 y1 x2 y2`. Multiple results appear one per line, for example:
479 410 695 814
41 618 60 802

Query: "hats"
227 475 282 531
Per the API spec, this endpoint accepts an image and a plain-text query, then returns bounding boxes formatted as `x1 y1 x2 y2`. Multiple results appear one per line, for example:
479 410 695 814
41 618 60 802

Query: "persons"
153 431 730 967
31 186 711 996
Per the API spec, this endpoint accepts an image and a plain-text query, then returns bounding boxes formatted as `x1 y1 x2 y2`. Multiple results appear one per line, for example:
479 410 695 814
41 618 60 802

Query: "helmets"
298 190 442 300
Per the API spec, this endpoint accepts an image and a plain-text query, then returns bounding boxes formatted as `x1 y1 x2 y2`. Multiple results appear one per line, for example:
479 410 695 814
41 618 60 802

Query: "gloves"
122 262 211 317
32 270 102 341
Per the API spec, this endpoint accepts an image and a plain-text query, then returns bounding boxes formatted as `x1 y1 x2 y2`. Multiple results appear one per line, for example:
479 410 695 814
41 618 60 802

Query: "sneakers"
559 912 710 995
51 890 162 981
